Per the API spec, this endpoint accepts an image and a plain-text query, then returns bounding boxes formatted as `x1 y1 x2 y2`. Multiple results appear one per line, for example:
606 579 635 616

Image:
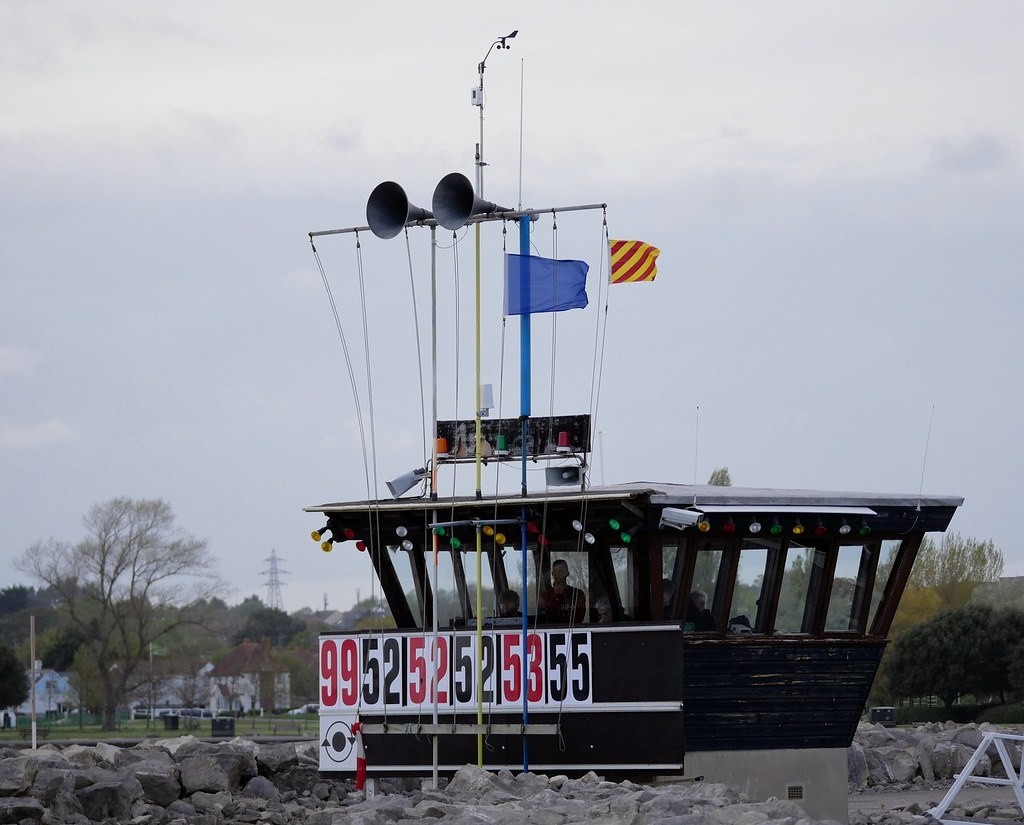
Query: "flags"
609 240 660 285
502 252 588 317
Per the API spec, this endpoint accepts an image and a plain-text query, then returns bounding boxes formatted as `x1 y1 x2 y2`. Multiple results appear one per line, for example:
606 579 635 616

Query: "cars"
287 704 320 716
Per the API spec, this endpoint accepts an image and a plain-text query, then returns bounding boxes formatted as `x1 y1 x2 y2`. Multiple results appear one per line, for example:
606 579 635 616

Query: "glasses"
598 612 608 617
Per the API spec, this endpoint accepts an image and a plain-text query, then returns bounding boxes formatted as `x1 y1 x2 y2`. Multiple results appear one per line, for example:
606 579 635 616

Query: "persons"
495 590 523 618
682 589 717 632
537 558 587 624
728 614 752 634
661 576 675 617
595 595 632 623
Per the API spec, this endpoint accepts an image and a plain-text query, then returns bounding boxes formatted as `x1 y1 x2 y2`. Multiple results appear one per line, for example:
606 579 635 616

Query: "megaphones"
432 172 514 231
366 180 434 239
545 464 583 487
386 470 418 498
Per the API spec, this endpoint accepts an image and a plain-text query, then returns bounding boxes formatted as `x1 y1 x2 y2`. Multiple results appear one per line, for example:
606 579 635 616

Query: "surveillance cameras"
662 507 704 524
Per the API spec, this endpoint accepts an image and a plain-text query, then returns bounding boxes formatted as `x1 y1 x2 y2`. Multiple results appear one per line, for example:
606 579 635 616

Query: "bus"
869 705 897 728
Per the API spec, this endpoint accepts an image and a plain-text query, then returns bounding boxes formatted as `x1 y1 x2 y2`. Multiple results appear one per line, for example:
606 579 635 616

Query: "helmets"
497 590 520 611
